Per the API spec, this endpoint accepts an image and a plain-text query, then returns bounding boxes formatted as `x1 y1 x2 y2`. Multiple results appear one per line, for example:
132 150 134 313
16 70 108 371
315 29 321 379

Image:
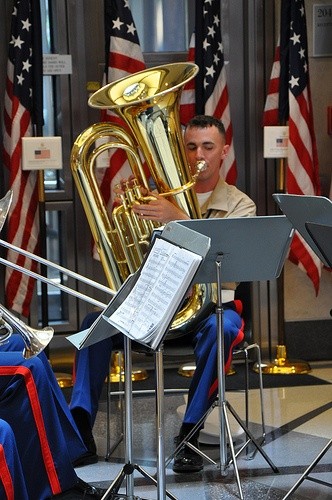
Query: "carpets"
60 361 332 403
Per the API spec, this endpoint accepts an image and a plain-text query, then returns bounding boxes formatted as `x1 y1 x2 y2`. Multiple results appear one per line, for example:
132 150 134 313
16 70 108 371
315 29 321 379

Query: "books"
109 234 203 351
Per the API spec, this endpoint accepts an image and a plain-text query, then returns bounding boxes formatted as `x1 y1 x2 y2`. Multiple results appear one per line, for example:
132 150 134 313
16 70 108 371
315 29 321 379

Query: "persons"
70 116 256 473
0 334 113 500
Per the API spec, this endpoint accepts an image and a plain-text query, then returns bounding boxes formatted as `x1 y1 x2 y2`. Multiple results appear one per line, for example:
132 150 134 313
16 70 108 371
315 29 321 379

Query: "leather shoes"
172 436 204 471
53 476 115 500
72 437 98 467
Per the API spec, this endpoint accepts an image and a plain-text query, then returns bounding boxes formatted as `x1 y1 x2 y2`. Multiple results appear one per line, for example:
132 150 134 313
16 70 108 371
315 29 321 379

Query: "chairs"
105 280 265 460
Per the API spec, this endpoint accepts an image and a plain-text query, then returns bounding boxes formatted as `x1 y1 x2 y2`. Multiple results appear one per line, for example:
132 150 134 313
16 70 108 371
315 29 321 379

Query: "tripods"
164 214 296 499
80 275 176 500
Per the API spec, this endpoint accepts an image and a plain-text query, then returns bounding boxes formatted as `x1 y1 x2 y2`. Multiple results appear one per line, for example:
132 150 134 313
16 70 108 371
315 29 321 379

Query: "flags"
0 0 41 317
178 0 238 186
92 0 146 262
263 0 323 296
